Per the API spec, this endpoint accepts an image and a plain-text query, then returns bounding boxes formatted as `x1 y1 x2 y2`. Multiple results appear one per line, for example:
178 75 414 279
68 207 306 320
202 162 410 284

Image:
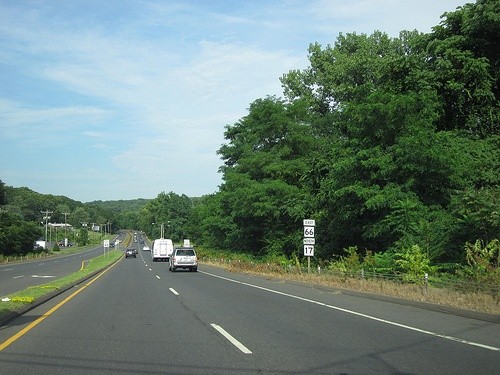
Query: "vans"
150 238 174 262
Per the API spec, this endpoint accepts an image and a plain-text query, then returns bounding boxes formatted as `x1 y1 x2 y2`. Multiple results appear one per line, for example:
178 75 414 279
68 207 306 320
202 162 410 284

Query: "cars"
134 232 151 252
125 248 136 258
33 244 43 253
58 240 73 247
109 243 114 247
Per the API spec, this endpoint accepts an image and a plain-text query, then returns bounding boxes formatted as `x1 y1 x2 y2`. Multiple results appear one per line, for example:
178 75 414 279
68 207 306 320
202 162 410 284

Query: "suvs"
168 247 198 272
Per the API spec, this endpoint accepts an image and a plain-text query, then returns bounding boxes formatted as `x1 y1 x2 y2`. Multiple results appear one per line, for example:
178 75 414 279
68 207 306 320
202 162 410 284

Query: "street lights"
152 222 162 238
162 220 170 239
